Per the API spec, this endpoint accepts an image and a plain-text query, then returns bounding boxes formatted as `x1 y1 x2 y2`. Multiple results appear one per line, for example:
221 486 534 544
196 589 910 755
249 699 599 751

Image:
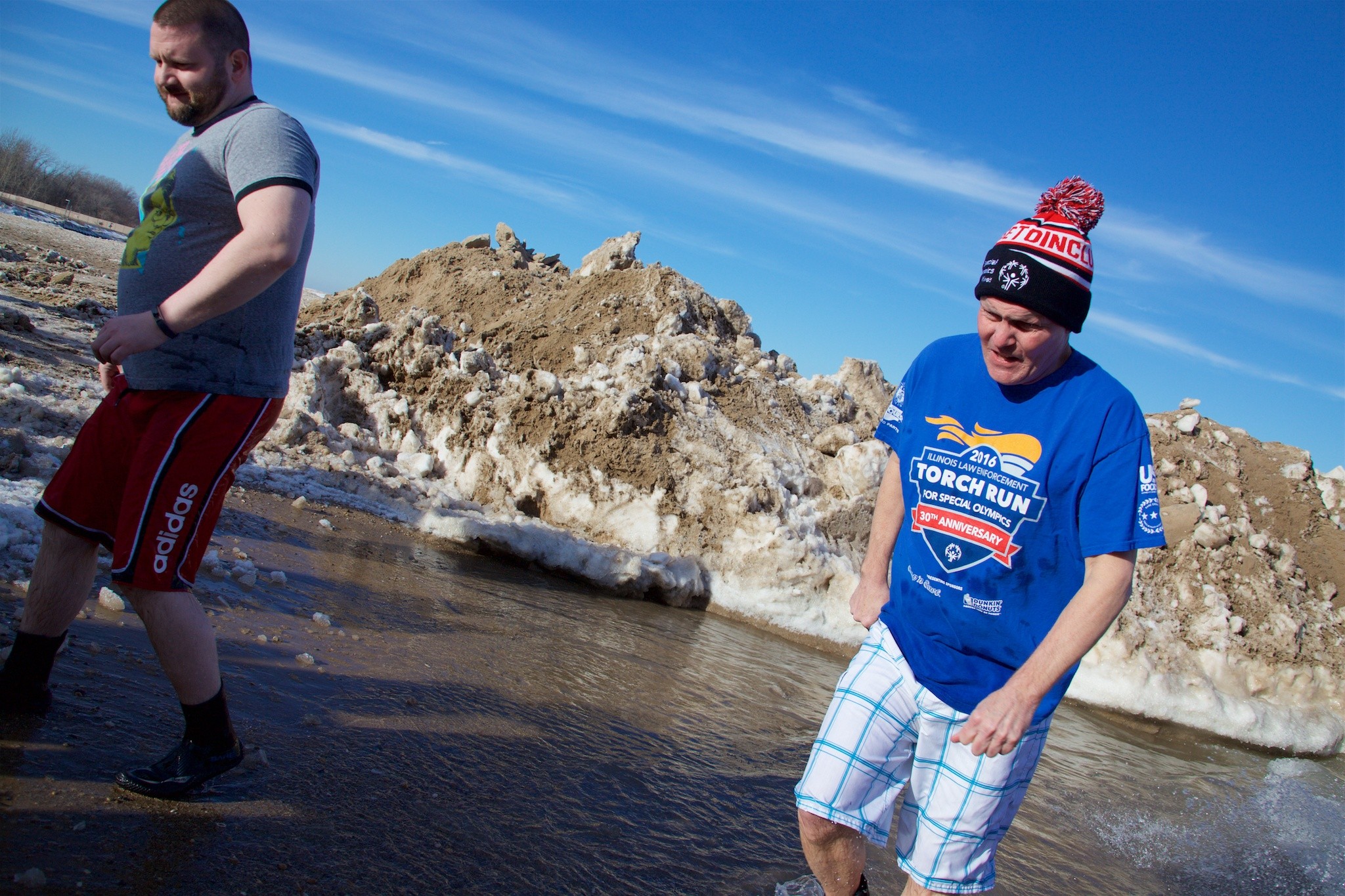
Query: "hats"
974 175 1107 335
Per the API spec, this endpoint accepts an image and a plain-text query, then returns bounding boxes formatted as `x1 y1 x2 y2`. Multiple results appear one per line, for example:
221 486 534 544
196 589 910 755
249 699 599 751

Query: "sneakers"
0 670 55 719
115 741 245 795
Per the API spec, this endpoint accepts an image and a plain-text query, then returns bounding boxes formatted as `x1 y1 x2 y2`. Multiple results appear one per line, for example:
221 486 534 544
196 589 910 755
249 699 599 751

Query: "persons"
150 305 180 338
0 2 321 793
791 180 1169 896
